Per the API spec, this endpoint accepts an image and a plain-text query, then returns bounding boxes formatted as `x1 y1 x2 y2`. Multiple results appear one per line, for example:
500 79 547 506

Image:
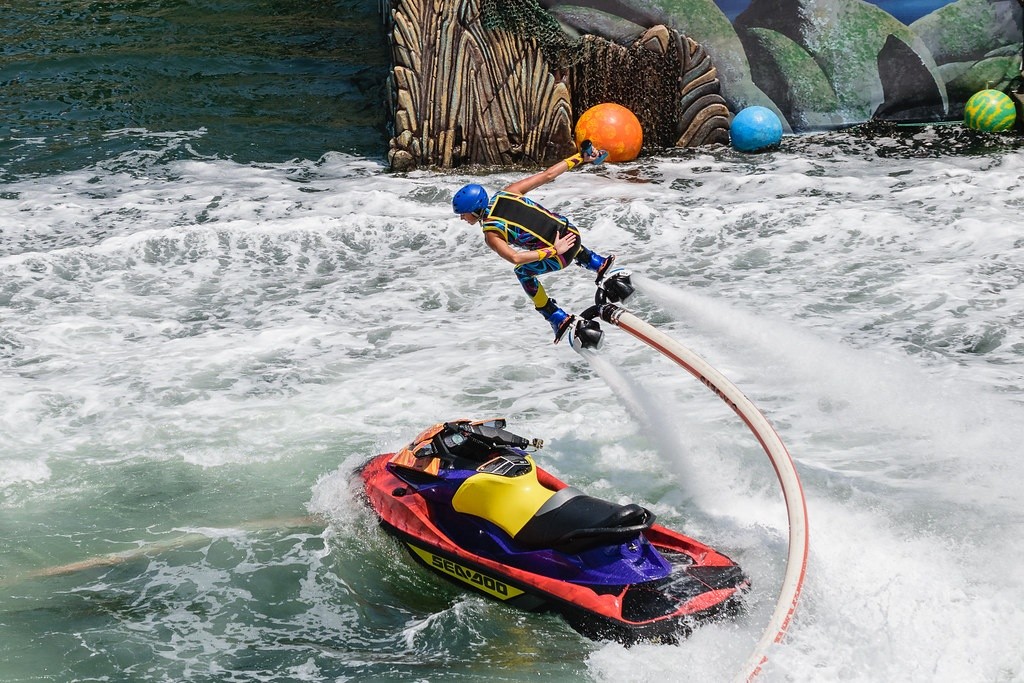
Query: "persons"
452 145 614 344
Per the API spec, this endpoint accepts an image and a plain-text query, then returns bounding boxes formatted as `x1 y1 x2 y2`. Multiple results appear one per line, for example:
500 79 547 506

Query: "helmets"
452 184 489 215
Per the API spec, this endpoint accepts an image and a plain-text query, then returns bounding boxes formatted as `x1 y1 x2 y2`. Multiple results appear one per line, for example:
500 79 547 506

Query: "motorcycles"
347 417 752 648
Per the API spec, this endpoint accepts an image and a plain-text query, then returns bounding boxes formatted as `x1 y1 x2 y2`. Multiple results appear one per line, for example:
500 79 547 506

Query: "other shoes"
579 251 606 273
547 308 570 336
